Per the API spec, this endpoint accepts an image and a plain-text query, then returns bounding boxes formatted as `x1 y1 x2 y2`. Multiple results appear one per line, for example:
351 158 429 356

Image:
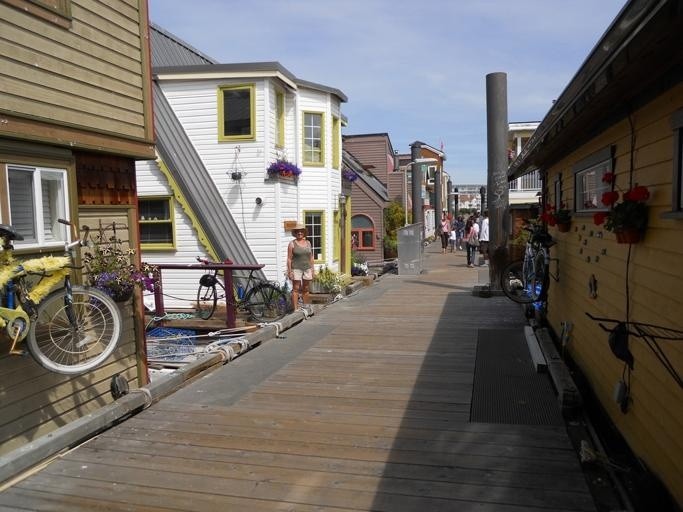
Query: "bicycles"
499 216 556 305
0 218 121 374
195 256 288 324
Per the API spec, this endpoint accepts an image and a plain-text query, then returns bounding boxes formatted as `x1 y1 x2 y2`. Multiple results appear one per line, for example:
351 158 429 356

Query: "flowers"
539 202 571 226
590 172 650 231
81 235 159 310
267 161 301 177
341 170 356 181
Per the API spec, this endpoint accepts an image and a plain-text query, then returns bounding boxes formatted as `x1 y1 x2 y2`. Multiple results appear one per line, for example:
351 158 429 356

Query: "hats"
291 224 309 237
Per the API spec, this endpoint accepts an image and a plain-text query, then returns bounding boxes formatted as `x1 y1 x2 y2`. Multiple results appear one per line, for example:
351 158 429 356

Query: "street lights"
404 157 438 227
444 192 460 214
338 195 346 276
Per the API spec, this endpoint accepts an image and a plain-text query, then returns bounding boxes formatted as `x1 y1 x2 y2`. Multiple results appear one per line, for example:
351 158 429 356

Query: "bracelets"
287 271 292 275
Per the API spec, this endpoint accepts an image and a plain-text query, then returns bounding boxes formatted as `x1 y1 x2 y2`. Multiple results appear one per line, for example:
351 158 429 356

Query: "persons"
284 223 314 313
437 209 489 267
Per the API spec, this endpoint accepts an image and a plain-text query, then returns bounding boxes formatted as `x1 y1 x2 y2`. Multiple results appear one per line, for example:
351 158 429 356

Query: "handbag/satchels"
467 228 480 247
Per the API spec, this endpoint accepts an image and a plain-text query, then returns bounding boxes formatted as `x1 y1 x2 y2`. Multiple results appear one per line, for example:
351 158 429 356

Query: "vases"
557 221 569 232
614 224 644 244
